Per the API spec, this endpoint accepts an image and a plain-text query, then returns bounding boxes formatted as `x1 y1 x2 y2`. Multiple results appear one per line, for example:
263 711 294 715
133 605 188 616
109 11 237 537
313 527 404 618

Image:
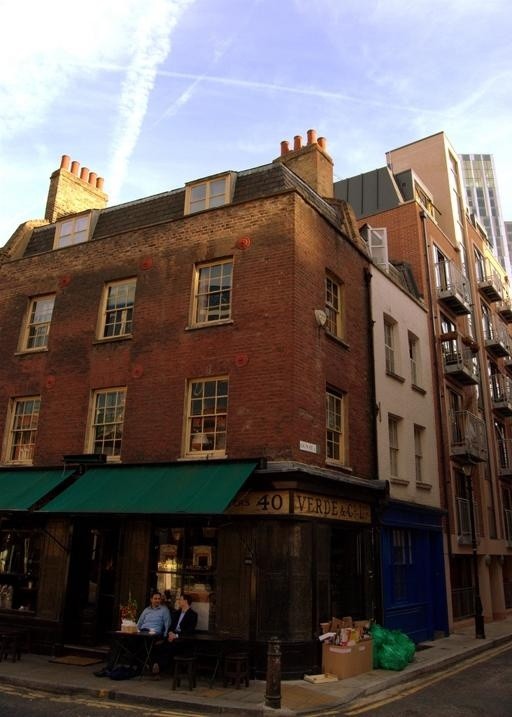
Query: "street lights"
453 454 487 639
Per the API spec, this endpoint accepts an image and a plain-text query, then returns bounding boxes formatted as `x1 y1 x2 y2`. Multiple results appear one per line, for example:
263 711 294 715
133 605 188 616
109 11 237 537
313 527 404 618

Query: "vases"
121 623 138 633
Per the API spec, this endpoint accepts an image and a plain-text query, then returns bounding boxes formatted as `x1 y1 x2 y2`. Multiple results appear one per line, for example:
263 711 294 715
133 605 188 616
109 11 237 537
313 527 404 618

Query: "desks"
110 630 159 681
179 633 230 689
0 606 36 658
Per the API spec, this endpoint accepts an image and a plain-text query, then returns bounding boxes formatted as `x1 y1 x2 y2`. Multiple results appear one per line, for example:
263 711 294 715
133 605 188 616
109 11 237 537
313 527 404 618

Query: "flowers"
120 587 138 622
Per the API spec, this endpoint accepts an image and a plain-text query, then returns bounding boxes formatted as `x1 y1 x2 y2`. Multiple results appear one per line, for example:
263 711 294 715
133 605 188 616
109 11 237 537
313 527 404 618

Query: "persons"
136 591 172 681
152 595 197 674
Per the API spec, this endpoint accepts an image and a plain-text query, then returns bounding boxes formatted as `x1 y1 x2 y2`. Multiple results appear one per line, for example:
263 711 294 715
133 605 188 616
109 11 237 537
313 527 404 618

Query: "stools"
174 656 197 691
0 627 18 663
222 654 249 691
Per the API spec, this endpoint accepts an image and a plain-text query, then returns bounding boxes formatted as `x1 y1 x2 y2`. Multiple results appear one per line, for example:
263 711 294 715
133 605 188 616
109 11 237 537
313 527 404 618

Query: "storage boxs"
322 640 373 680
